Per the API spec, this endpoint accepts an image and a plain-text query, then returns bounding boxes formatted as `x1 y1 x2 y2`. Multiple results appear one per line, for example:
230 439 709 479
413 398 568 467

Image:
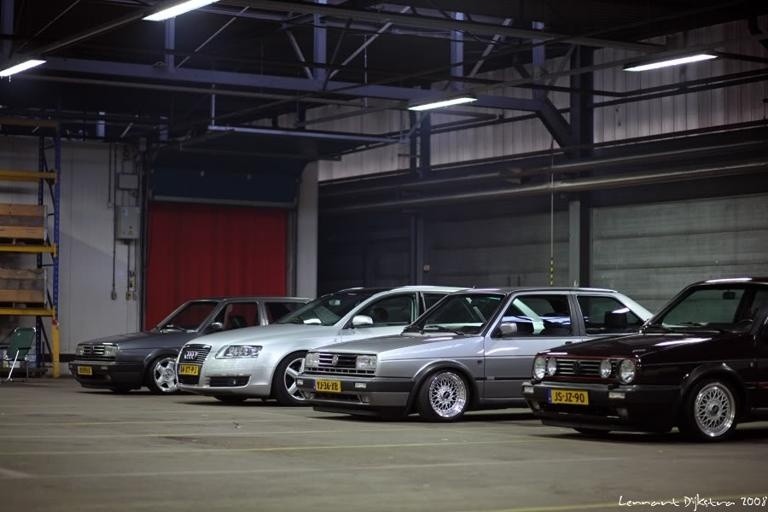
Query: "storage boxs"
0 204 49 306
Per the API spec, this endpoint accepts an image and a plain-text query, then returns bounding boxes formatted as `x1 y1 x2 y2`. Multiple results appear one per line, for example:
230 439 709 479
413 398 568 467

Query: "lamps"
0 0 220 79
622 45 719 72
407 90 479 112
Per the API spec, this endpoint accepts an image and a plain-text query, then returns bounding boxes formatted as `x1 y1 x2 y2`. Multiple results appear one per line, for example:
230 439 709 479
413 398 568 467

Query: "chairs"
229 316 246 330
496 311 627 335
0 327 36 381
370 307 389 323
